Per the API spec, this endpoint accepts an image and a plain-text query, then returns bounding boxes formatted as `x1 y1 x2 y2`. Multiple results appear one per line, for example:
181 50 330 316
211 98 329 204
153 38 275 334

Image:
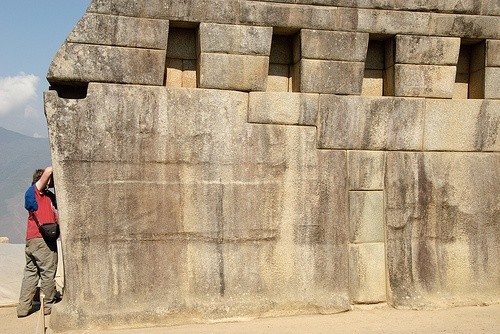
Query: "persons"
16 166 58 318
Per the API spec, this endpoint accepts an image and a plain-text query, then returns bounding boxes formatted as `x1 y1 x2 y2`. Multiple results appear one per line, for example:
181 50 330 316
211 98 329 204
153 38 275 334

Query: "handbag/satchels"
40 223 60 239
